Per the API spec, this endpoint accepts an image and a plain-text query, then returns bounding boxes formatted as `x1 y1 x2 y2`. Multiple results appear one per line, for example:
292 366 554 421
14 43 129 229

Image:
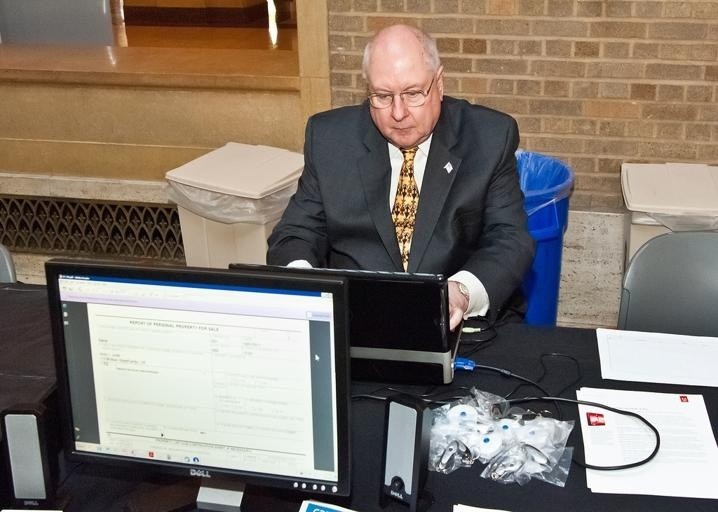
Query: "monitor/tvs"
45 259 349 512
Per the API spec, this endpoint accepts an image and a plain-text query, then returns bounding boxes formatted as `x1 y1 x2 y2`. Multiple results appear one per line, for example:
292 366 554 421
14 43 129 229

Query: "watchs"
454 280 471 302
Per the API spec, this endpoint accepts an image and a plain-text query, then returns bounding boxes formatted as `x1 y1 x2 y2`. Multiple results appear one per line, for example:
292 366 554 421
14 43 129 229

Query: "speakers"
0 402 54 509
381 394 432 512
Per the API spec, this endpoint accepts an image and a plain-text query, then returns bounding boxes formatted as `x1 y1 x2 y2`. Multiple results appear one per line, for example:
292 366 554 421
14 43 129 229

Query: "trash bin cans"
165 142 305 270
621 163 718 270
515 151 573 326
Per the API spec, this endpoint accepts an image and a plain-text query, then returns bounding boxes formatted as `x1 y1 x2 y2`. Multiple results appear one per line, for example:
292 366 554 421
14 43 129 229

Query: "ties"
392 146 420 273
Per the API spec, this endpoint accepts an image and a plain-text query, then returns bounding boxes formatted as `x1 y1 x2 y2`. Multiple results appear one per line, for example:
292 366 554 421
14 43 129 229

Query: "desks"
0 283 718 512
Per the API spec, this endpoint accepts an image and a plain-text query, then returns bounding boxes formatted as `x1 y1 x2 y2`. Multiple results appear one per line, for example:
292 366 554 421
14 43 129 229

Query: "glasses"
366 64 441 109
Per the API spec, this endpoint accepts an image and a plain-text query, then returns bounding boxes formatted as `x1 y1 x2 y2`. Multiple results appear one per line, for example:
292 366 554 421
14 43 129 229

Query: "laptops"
229 261 465 386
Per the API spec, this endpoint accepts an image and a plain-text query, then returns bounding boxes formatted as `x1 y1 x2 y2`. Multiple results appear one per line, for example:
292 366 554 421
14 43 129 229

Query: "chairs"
616 229 718 338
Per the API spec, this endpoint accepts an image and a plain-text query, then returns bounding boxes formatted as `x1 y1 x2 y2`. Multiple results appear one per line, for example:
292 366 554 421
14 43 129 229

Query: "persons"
265 23 537 336
